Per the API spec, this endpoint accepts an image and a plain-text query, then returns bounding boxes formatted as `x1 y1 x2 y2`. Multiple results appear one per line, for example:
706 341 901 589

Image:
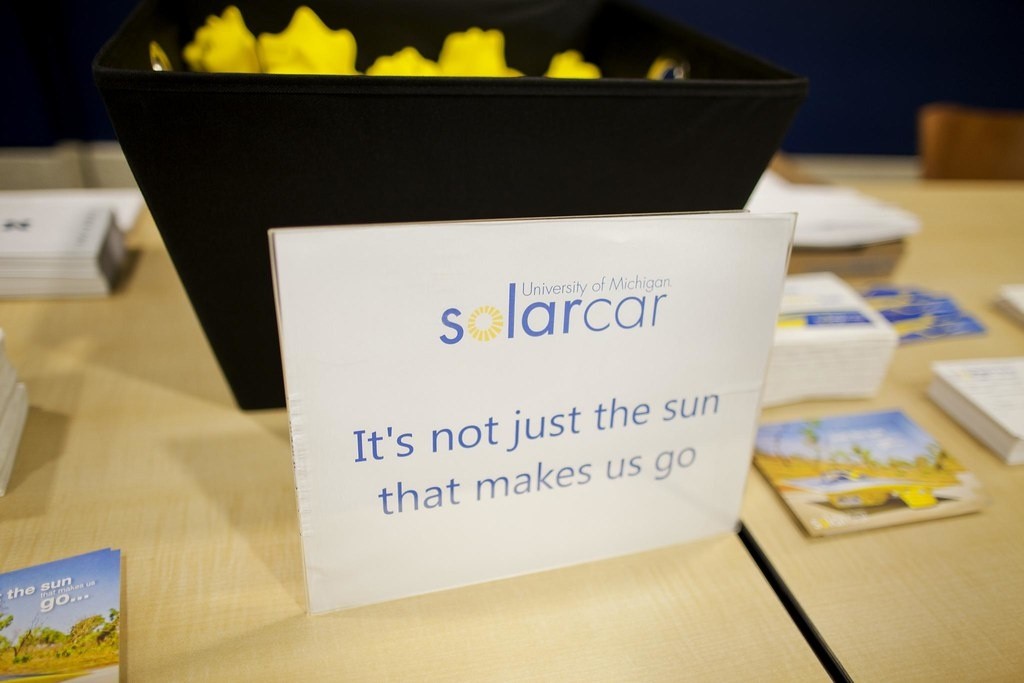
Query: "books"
750 172 918 277
0 187 142 300
754 410 984 537
760 272 899 408
923 356 1024 465
0 547 121 683
0 330 30 501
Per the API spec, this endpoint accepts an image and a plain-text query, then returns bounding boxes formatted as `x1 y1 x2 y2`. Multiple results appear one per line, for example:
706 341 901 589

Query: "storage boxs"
90 0 803 410
